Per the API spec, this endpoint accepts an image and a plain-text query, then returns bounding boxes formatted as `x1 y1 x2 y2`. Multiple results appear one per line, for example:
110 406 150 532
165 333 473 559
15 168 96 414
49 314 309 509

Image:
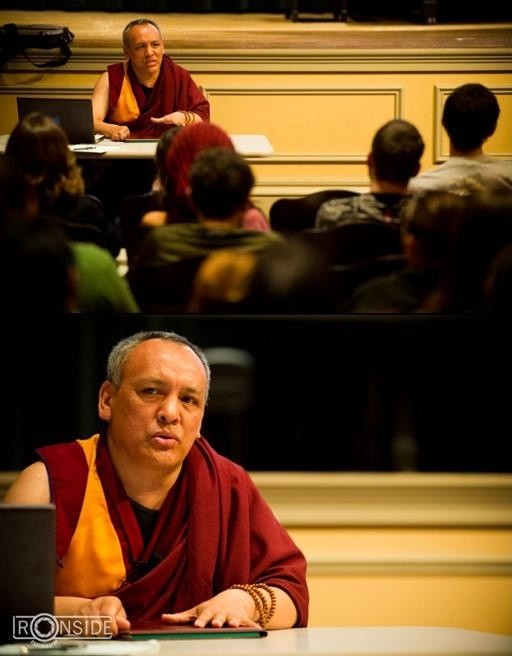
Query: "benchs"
3 190 511 469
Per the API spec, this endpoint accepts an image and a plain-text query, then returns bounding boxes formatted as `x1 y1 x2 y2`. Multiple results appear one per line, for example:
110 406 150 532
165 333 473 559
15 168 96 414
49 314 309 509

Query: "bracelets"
230 582 277 629
180 110 196 125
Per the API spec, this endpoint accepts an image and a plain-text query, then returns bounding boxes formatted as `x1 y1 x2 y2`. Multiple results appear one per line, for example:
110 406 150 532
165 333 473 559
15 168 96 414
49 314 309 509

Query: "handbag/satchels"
0 22 75 48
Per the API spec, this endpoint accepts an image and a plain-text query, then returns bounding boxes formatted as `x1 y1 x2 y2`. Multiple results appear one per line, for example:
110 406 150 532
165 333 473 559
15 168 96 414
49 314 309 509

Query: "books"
121 623 268 642
116 130 168 142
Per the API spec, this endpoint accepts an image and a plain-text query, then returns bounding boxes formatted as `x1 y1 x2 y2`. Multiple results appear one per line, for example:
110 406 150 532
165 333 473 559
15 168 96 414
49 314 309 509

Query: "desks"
1 130 274 160
0 625 510 656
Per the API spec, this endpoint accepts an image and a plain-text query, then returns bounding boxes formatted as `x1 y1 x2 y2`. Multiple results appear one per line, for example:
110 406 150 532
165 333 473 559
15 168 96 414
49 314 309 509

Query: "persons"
4 332 309 639
91 18 209 140
2 83 512 471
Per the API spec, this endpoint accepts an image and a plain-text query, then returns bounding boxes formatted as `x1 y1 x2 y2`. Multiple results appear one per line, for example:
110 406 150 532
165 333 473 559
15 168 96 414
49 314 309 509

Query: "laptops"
0 505 64 645
17 96 105 144
